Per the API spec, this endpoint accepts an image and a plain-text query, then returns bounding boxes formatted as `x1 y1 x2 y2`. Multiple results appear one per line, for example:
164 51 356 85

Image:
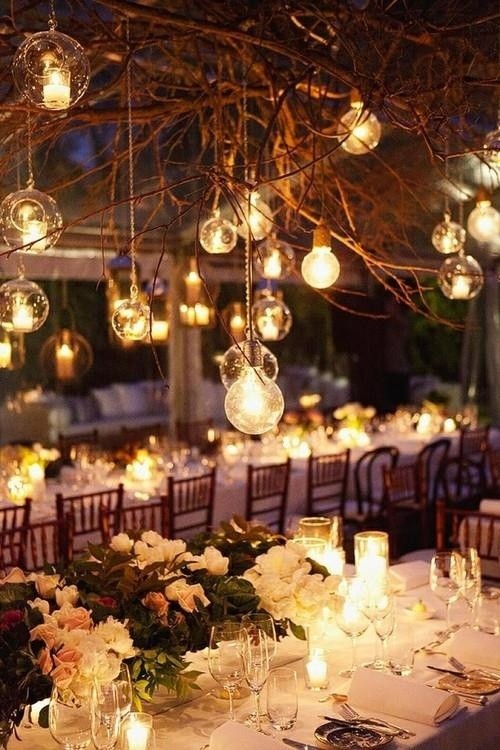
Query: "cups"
50 547 499 750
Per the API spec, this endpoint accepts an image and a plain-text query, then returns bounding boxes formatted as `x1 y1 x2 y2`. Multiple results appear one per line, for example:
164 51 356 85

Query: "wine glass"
287 516 389 591
1 402 482 504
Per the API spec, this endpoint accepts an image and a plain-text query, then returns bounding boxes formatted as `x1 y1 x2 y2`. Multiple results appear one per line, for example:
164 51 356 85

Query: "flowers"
0 511 346 750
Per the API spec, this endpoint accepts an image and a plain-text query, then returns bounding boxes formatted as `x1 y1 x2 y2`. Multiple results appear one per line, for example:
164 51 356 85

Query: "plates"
315 665 499 749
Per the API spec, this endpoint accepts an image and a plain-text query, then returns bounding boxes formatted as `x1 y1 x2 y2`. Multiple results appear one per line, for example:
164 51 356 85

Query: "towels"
210 718 296 750
344 666 461 725
445 625 500 666
